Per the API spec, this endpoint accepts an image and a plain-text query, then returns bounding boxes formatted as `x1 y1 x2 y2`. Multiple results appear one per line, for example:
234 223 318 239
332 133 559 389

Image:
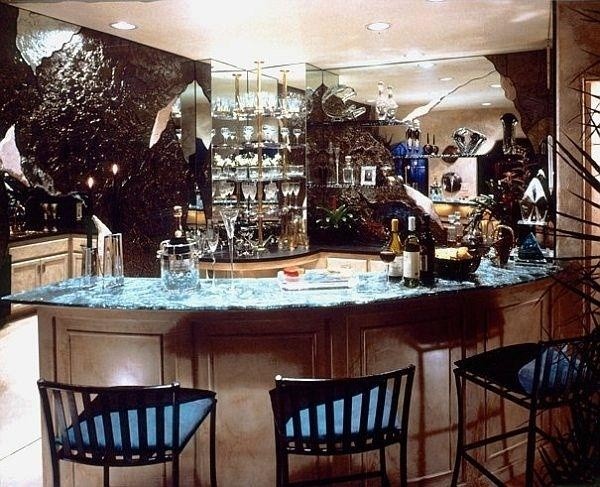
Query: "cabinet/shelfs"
198 58 310 260
306 111 486 253
8 229 98 315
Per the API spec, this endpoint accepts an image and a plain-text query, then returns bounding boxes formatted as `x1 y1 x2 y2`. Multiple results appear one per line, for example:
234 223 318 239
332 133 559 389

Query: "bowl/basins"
158 251 199 292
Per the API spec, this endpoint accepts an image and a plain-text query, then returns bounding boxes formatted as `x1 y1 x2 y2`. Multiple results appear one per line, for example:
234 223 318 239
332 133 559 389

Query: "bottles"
446 215 456 243
421 213 435 285
342 156 355 184
402 217 420 289
454 210 463 243
387 219 402 283
167 206 189 260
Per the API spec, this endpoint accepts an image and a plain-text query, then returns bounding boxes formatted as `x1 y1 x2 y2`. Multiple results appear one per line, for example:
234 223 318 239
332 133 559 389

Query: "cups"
380 247 396 291
250 183 257 202
294 182 301 209
230 182 235 207
207 230 220 293
288 182 294 208
242 182 249 201
223 183 230 208
281 183 289 208
219 208 243 295
219 185 225 207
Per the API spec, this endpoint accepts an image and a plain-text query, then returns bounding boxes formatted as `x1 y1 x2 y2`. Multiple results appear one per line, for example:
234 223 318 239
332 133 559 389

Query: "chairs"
267 362 416 487
37 379 217 486
452 329 600 487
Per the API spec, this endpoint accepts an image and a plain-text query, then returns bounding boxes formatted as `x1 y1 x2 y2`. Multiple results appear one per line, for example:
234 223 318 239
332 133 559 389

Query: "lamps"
366 22 392 31
110 21 139 30
